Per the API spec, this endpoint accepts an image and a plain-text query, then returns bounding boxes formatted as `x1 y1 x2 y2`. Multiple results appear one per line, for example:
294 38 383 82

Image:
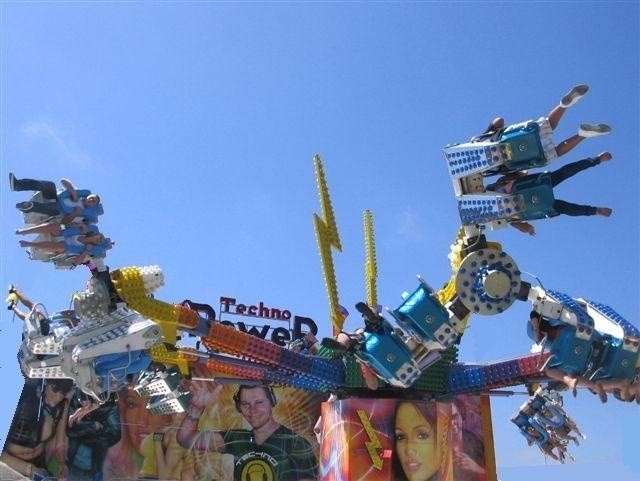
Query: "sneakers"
561 84 589 108
578 123 612 138
8 284 15 310
558 432 585 464
15 202 35 210
8 172 17 190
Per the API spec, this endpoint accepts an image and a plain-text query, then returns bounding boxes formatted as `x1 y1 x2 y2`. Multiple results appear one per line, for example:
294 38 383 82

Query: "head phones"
233 383 278 413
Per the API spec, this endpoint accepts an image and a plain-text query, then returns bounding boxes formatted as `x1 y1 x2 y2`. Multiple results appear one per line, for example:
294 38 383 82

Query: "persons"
474 83 612 171
521 311 640 464
176 381 319 481
5 373 184 481
483 151 613 237
9 167 106 262
387 399 485 481
323 303 380 352
6 282 52 334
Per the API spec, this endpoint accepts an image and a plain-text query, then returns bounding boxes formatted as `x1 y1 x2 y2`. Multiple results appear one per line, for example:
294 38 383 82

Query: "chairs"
24 190 187 416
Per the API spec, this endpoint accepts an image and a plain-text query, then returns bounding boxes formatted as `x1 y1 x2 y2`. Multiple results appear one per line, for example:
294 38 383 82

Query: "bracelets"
187 410 204 421
